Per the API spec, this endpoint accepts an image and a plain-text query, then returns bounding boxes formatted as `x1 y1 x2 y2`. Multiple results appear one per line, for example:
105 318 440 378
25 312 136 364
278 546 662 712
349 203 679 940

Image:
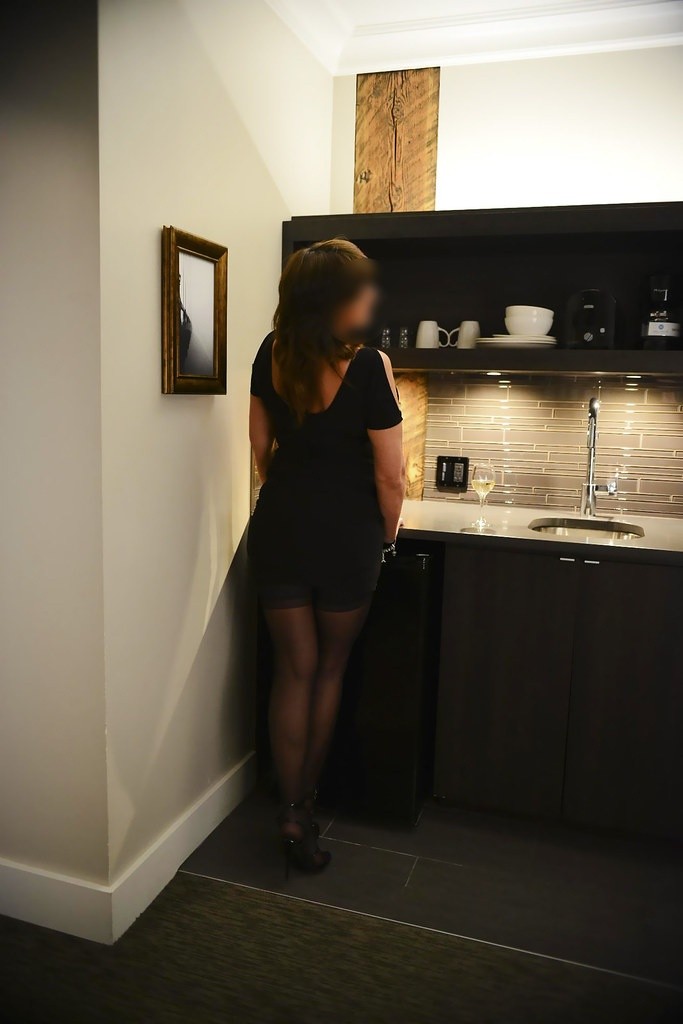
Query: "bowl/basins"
505 306 555 317
504 317 553 335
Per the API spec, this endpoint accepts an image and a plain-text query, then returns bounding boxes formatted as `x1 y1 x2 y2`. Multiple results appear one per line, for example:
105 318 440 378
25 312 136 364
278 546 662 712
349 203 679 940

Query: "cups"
449 321 482 350
415 321 449 348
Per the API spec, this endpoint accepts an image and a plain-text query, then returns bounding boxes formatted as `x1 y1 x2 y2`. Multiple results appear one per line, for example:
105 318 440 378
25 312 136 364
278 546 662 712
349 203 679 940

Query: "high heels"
274 800 333 880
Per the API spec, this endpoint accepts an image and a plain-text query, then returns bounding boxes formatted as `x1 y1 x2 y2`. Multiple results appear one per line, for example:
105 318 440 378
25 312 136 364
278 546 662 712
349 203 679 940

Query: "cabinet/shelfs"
431 547 683 846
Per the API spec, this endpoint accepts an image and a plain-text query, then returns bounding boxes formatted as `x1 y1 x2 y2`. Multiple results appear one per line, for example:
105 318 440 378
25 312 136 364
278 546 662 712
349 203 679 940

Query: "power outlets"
436 455 468 493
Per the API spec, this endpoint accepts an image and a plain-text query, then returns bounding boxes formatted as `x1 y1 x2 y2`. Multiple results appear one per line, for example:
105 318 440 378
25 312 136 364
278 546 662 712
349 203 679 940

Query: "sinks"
527 517 645 541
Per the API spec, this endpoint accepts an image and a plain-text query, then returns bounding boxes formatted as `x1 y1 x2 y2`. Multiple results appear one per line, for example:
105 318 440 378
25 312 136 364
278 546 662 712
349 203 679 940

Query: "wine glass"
471 464 496 527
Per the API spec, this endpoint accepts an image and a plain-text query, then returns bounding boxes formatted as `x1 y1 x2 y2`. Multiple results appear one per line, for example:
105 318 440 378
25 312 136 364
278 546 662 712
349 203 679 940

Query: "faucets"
581 398 618 514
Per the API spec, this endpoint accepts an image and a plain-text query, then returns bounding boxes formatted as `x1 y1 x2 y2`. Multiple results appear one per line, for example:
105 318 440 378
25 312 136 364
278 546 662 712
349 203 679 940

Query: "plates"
475 334 558 349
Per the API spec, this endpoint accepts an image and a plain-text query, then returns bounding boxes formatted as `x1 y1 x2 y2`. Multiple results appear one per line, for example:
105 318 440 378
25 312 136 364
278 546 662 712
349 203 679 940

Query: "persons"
245 238 405 883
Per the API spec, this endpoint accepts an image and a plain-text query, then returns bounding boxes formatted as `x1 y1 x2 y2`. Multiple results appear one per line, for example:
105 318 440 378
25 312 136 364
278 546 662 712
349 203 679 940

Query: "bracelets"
381 537 398 563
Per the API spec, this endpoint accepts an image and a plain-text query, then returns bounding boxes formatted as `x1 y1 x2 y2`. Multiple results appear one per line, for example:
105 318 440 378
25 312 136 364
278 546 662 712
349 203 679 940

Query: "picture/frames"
160 226 229 401
